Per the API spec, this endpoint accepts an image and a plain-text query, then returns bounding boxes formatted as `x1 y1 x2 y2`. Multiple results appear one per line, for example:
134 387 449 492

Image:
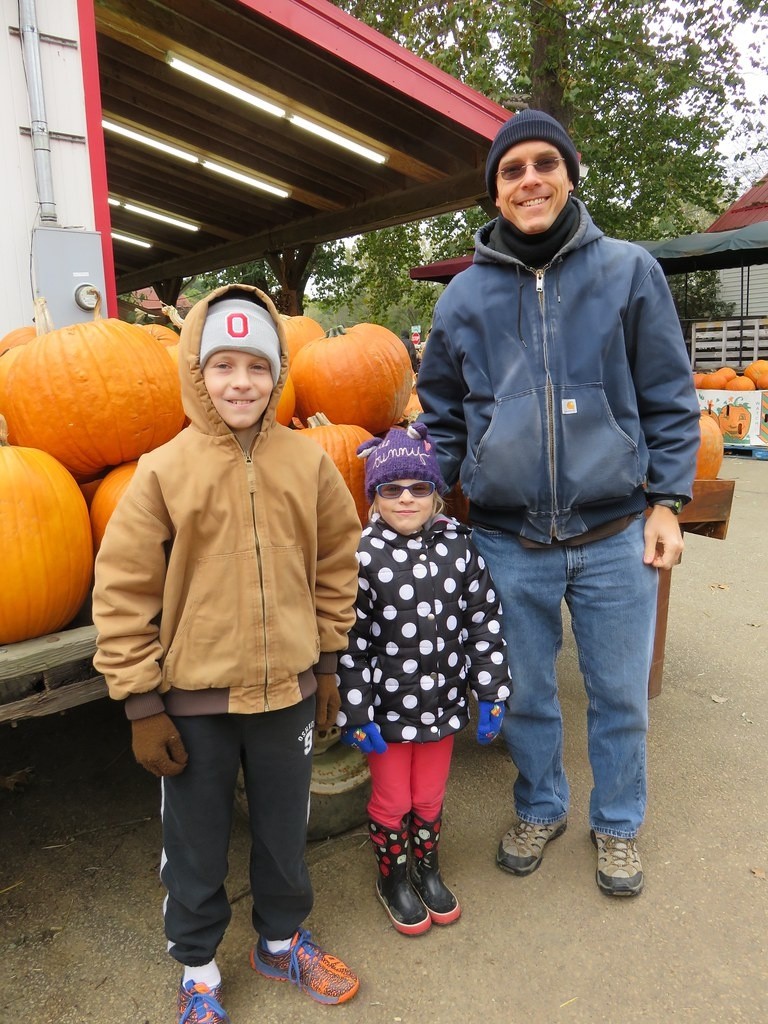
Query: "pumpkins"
0 289 468 647
695 414 724 480
692 358 768 391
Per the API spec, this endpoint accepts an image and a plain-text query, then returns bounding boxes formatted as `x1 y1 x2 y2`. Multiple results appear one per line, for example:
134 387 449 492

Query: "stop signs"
412 332 420 345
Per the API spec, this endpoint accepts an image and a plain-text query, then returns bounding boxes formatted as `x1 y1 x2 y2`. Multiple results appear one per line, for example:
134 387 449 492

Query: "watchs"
653 498 683 514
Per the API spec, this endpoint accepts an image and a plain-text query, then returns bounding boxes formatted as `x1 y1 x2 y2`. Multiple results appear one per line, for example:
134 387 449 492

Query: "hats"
484 108 579 204
200 299 281 388
357 423 451 503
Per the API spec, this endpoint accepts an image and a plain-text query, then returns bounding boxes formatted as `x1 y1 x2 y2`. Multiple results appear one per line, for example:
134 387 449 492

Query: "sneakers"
177 974 230 1024
250 927 360 1004
589 829 644 895
497 814 567 875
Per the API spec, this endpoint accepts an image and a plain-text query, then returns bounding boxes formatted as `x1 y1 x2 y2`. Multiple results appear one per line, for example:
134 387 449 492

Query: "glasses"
493 155 567 180
374 482 437 499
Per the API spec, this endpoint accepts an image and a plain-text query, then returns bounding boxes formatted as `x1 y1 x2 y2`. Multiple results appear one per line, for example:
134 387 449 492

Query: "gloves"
130 709 188 777
340 722 386 754
476 702 505 744
315 672 340 730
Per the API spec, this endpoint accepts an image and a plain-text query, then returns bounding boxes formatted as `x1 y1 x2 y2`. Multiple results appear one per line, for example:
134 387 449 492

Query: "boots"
406 810 461 924
367 815 431 935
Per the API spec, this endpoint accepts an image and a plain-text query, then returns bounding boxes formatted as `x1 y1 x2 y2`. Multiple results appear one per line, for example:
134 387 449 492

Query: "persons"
416 109 700 895
92 284 363 1024
336 423 513 937
400 329 418 374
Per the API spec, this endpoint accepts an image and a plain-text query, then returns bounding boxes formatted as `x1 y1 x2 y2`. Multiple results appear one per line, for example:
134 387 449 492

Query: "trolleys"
1 604 374 847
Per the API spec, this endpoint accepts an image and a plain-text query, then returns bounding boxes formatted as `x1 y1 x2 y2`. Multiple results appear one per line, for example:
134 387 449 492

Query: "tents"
410 246 475 284
631 221 768 366
